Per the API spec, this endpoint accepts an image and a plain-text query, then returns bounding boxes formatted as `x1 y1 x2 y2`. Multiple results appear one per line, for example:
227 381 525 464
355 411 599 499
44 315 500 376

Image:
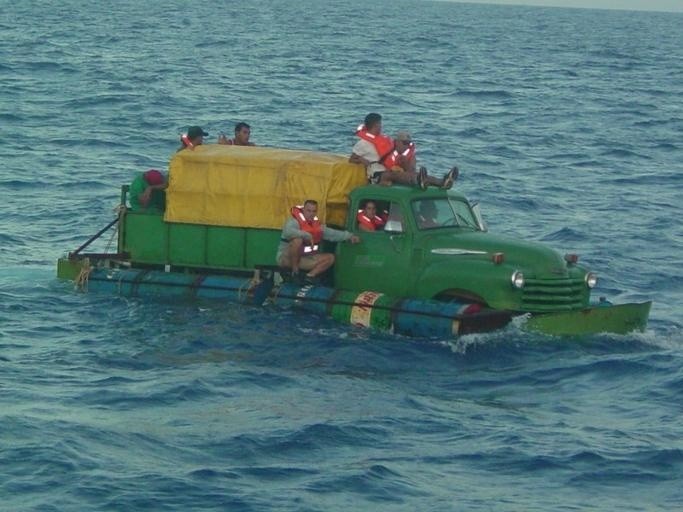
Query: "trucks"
54 145 654 344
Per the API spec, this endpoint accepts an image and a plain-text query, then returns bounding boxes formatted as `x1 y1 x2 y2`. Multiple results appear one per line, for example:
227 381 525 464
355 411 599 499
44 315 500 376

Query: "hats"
187 125 210 137
145 169 163 186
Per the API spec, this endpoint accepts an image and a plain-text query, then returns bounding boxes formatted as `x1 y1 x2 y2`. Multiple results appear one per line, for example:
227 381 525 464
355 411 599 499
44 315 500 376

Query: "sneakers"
417 166 428 191
290 271 305 287
442 166 459 190
305 274 319 287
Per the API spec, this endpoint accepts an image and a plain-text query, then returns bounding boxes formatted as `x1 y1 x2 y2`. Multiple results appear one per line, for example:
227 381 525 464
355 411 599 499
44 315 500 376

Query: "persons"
217 121 256 147
348 112 460 232
275 198 361 289
176 126 209 153
129 169 169 215
